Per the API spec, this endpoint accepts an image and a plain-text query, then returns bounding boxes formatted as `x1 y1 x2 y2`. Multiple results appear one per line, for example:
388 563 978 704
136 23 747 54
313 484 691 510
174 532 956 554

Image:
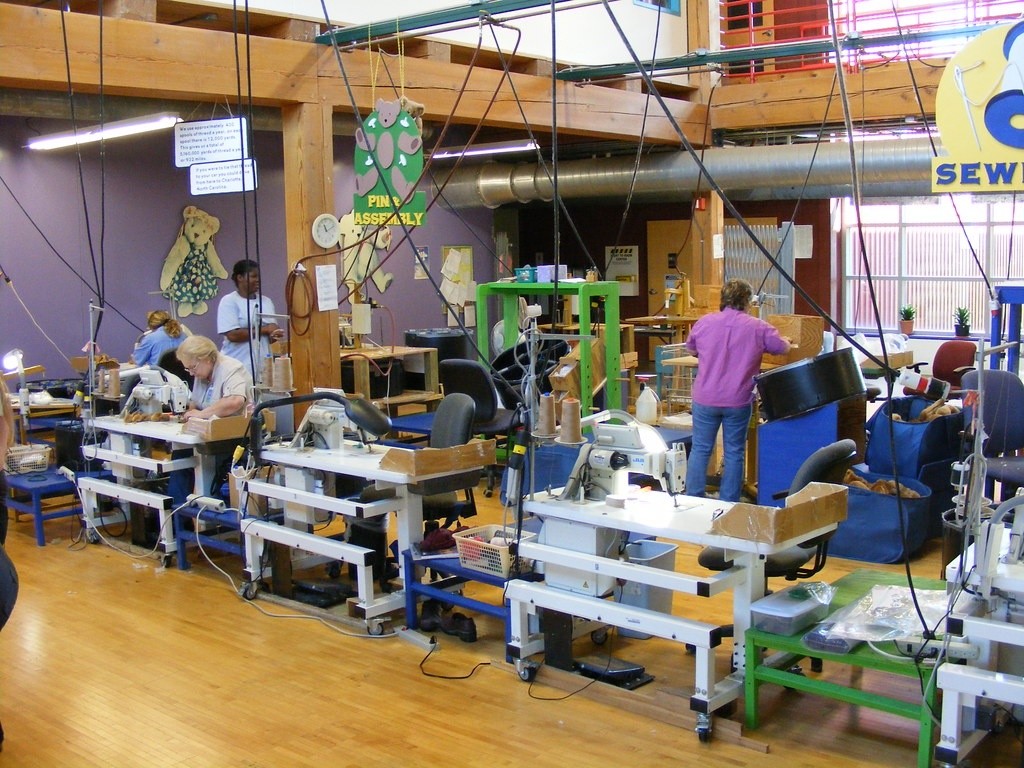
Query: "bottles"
635 387 657 426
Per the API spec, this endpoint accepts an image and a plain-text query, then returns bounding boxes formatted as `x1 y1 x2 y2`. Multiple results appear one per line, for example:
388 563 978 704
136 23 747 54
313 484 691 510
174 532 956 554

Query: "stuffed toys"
160 206 228 317
338 208 393 304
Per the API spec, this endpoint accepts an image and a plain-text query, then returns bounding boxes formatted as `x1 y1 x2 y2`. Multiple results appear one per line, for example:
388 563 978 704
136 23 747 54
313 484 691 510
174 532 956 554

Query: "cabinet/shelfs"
338 279 723 461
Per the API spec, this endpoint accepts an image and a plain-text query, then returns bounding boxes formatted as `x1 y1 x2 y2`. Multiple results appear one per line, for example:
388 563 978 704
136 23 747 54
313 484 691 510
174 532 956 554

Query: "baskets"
453 525 537 579
3 444 52 475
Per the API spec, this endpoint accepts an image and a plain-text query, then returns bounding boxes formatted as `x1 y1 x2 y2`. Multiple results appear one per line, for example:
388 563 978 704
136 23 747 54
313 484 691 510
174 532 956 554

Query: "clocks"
312 214 342 249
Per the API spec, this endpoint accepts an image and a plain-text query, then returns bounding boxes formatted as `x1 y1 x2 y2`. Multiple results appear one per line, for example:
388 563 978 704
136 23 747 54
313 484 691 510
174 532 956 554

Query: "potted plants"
900 306 914 334
955 308 972 336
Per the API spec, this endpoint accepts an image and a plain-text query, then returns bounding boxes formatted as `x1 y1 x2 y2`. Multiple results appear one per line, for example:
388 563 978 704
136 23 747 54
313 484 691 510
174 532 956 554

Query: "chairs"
900 341 1024 495
360 358 520 566
688 439 856 689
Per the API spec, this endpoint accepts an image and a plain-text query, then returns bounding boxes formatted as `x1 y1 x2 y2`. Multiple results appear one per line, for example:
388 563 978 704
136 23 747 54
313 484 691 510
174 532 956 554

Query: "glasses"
184 361 201 373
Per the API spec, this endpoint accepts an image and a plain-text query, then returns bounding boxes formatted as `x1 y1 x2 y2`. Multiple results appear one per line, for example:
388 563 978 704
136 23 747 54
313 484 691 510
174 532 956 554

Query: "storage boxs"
515 268 537 283
766 314 823 365
187 410 275 440
749 587 832 636
537 265 567 283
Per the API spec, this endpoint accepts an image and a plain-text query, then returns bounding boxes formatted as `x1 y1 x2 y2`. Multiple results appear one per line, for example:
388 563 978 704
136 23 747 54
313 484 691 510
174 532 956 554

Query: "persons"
216 260 284 381
128 310 187 367
685 277 793 502
150 335 256 540
0 374 19 750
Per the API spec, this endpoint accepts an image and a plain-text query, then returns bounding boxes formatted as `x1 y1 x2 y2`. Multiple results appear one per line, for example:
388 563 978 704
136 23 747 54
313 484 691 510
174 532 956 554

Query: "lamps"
21 111 184 149
421 139 538 159
2 347 30 427
250 393 393 469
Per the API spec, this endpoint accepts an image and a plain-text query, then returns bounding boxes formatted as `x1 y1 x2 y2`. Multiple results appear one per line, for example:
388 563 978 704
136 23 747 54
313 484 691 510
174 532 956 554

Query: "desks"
0 401 1024 768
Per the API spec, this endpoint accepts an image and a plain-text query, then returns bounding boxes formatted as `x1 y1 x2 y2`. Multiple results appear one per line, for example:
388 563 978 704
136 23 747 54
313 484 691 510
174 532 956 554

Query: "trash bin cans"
614 539 678 640
